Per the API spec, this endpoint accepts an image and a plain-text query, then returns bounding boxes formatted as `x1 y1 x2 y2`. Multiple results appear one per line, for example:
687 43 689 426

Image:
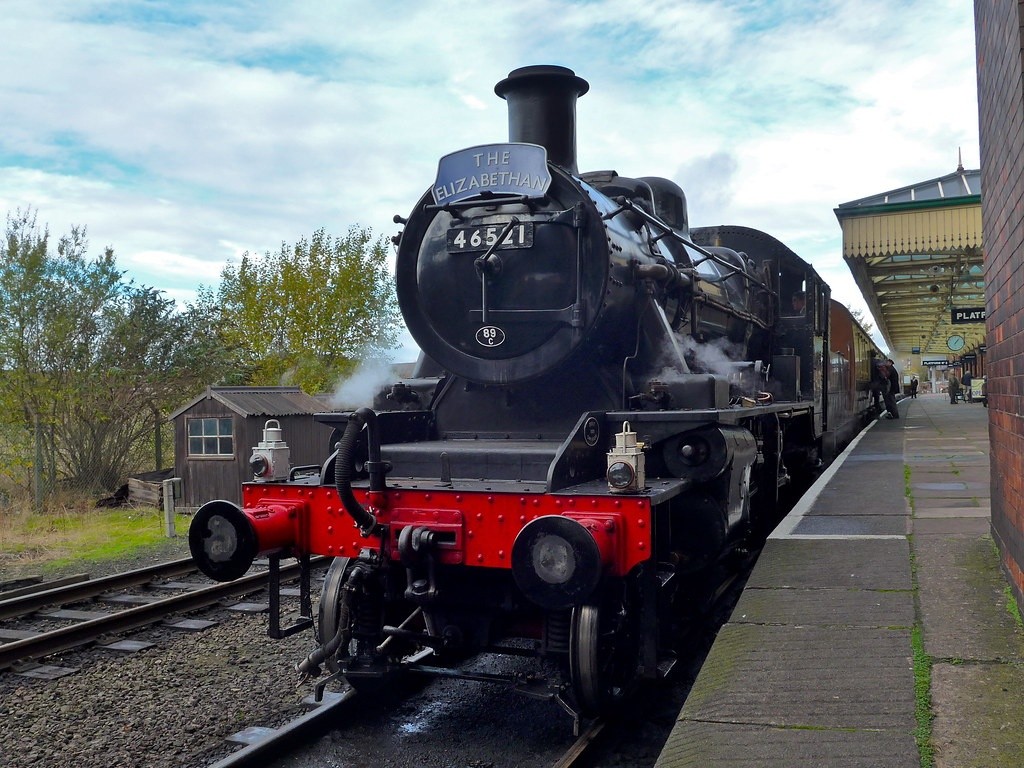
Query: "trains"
189 63 920 737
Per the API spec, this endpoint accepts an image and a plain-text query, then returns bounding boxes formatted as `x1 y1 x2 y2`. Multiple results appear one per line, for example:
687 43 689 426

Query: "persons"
869 349 883 420
791 291 806 317
981 375 988 408
961 371 975 401
947 368 960 405
884 358 901 419
910 376 919 399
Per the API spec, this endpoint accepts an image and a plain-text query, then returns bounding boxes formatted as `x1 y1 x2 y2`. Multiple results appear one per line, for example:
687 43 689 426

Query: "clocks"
947 335 965 351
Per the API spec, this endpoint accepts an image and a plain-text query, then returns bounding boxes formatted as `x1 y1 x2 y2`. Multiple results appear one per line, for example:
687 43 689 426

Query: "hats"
887 359 894 364
